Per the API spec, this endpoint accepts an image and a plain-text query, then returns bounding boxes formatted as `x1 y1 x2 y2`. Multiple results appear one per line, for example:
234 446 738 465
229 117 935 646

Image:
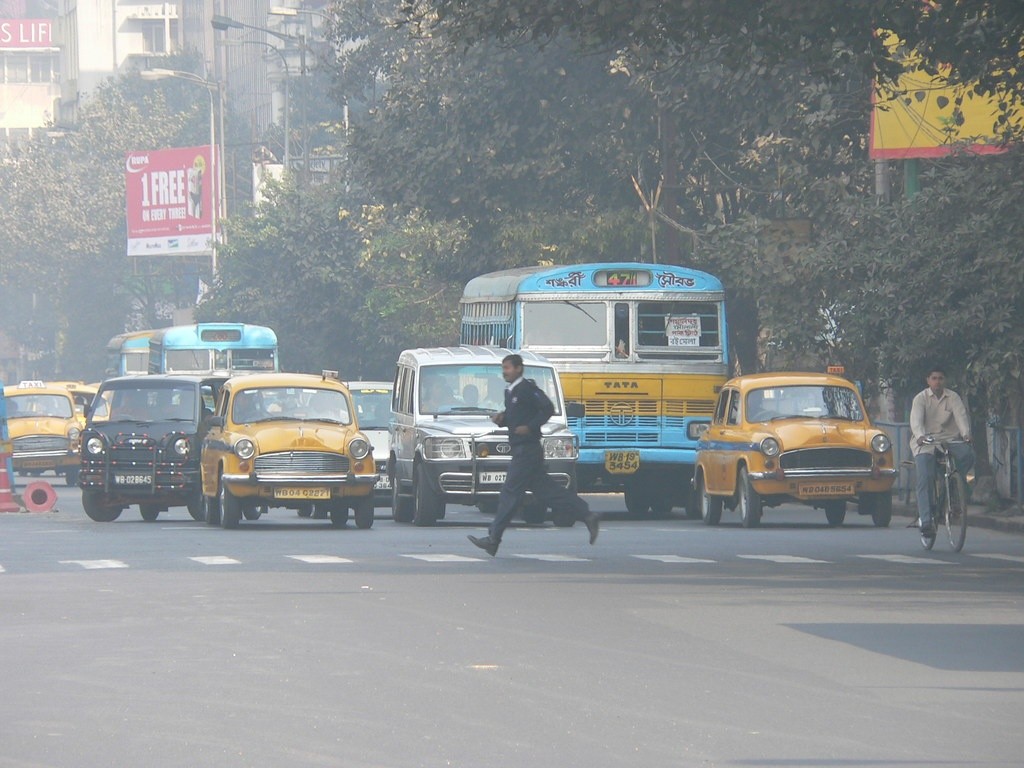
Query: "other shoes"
585 512 599 545
467 535 501 556
920 525 935 537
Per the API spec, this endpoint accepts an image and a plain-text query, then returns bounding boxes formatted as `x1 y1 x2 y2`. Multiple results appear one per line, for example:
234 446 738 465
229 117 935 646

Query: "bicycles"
899 436 978 553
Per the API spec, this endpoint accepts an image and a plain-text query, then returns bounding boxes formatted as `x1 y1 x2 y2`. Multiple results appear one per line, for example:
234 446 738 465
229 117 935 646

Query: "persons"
909 366 975 537
467 354 600 557
422 372 506 412
747 389 764 420
110 388 195 424
74 396 84 405
5 399 24 417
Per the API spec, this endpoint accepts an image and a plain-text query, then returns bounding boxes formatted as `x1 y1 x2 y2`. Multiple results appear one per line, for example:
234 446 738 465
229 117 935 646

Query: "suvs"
388 342 585 526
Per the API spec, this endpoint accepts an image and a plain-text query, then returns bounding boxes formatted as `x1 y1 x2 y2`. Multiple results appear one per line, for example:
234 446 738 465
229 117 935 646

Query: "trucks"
73 373 254 522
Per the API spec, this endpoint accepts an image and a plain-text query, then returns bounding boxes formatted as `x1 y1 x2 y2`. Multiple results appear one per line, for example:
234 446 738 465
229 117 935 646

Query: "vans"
310 381 422 501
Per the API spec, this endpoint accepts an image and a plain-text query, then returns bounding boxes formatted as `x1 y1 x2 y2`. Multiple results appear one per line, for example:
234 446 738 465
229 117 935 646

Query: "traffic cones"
19 480 59 514
0 446 20 513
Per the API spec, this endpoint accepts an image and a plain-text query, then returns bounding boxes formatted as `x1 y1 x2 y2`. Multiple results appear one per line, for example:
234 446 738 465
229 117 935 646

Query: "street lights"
268 7 350 199
217 38 291 198
152 66 217 296
138 68 231 243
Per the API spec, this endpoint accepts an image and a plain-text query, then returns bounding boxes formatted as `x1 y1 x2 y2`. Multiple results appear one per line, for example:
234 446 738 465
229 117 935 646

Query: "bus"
457 261 730 516
105 322 278 377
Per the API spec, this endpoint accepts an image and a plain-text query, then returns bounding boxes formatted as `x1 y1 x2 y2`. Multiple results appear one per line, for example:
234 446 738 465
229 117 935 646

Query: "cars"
689 371 897 527
4 376 117 486
198 369 382 528
